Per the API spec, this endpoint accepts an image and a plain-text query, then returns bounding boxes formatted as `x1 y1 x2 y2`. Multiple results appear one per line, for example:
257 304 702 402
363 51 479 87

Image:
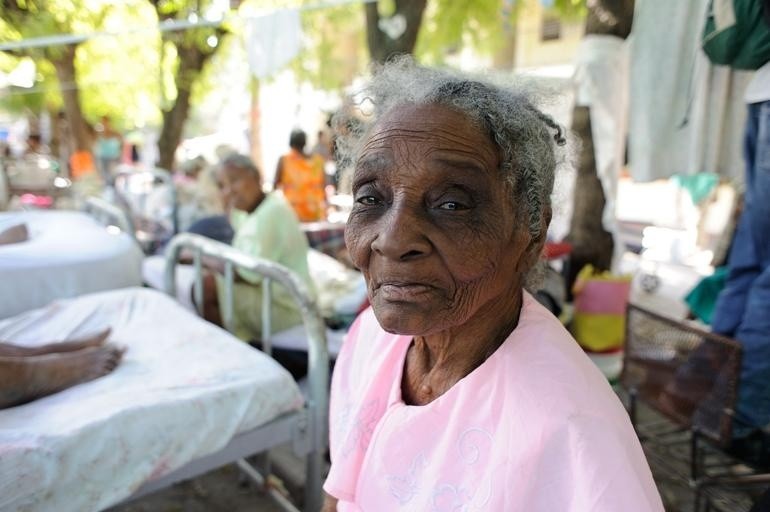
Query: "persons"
1 325 127 410
322 53 666 512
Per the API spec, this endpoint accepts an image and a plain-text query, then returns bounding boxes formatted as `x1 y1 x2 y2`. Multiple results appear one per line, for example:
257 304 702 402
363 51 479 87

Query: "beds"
0 194 146 319
1 231 330 511
